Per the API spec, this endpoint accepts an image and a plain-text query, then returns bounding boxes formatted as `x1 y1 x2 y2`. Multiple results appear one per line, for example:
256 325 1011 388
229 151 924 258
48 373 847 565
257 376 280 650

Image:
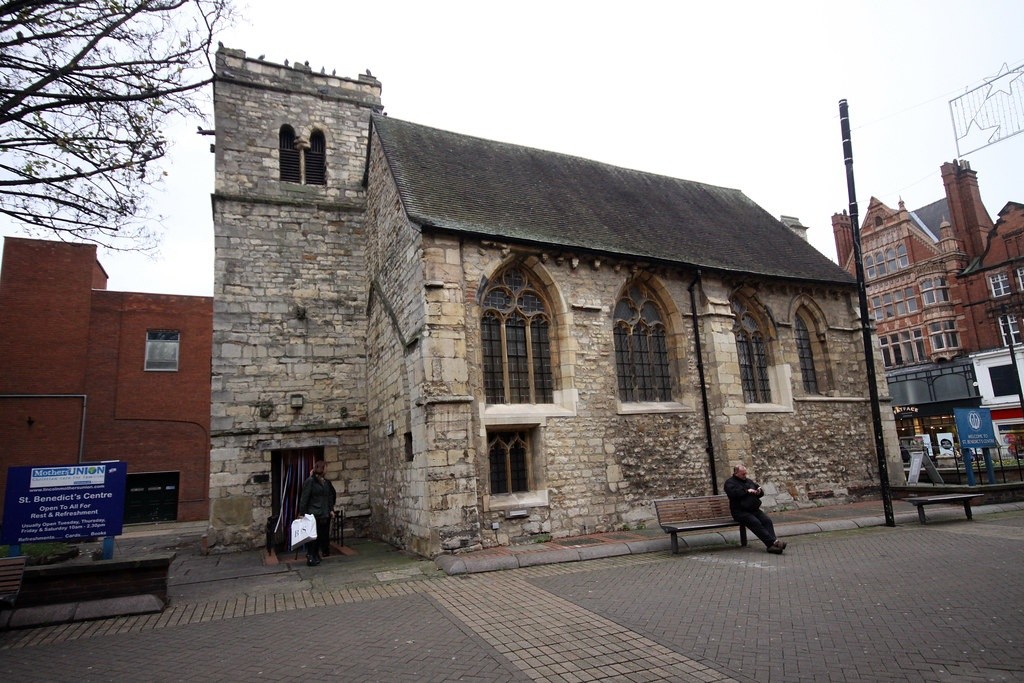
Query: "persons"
724 464 787 554
297 460 336 565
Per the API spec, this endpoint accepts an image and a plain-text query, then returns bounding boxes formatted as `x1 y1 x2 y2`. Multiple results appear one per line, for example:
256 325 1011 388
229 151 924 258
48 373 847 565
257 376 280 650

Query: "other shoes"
775 540 786 549
767 546 783 554
305 554 320 566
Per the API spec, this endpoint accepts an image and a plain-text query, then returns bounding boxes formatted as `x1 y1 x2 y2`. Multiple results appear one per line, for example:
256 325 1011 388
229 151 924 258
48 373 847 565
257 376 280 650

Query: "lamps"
290 393 304 411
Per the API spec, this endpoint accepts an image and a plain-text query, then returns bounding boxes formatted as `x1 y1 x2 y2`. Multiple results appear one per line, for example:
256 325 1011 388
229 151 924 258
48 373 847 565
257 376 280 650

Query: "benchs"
0 556 29 628
655 493 749 556
902 491 984 524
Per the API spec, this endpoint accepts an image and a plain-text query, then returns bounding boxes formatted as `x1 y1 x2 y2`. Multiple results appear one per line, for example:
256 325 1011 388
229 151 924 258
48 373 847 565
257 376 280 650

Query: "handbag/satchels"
290 514 318 551
